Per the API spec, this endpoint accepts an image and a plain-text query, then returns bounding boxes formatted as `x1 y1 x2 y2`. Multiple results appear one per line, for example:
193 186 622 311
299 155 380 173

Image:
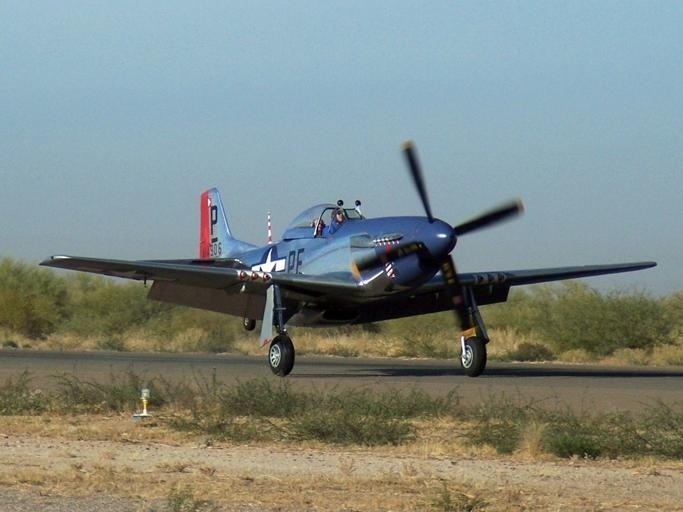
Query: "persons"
329 209 345 233
315 219 324 236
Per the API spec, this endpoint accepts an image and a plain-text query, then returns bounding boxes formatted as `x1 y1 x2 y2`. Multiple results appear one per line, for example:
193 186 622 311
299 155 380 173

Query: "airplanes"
35 141 662 380
